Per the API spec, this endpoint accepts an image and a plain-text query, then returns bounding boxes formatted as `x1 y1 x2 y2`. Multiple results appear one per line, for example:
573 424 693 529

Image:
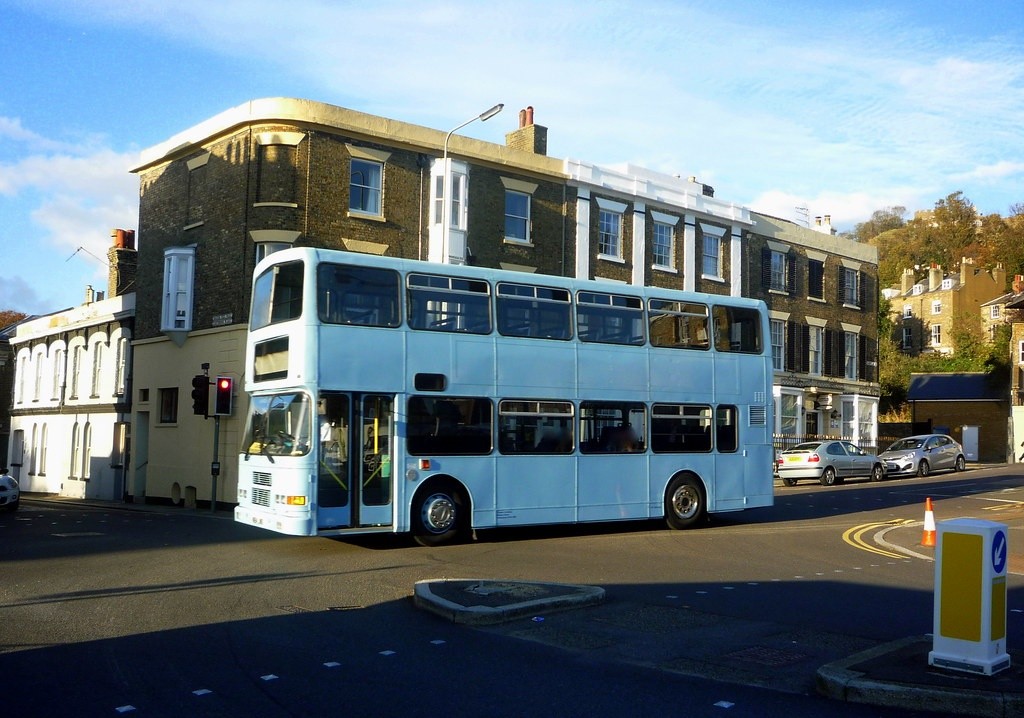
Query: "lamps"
802 407 806 416
831 408 841 419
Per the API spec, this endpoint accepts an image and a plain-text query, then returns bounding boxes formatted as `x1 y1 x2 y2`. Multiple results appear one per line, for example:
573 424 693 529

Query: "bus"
233 249 775 545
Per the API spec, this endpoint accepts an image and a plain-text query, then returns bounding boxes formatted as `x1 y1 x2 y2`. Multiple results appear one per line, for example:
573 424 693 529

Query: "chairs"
511 324 533 338
628 336 644 346
579 330 600 342
597 330 630 345
429 316 458 333
537 327 566 338
468 319 489 333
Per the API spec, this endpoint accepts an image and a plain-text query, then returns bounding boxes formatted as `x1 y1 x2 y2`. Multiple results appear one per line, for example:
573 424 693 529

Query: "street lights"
442 103 503 261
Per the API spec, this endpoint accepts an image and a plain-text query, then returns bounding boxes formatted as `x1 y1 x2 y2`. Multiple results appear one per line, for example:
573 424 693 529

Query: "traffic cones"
918 498 937 551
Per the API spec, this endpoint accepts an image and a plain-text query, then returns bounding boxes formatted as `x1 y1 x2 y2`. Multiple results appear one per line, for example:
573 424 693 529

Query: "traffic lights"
215 379 234 416
191 375 209 417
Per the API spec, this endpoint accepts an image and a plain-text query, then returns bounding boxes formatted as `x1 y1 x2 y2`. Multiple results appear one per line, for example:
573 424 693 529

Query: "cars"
0 469 20 514
879 434 966 478
778 438 886 487
